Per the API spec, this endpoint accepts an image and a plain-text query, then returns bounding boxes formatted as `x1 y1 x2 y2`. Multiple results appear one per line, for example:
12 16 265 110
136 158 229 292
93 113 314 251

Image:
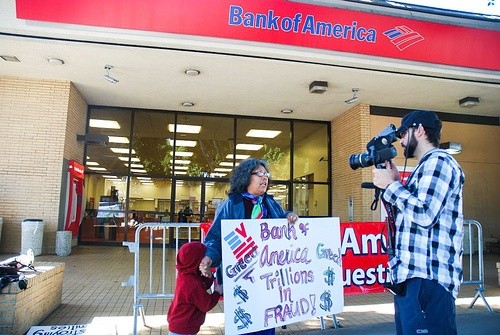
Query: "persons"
167 241 222 335
199 158 297 335
372 111 466 335
178 205 193 223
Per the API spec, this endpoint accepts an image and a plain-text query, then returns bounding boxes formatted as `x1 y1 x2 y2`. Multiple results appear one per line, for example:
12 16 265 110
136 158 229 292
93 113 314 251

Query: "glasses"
400 129 417 138
251 172 269 177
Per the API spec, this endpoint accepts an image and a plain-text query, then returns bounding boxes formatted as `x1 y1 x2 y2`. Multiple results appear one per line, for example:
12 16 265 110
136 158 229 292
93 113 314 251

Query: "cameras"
349 124 402 170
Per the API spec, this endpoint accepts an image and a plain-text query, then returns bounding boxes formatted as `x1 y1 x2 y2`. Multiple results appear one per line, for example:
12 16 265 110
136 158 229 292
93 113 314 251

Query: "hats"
394 111 441 136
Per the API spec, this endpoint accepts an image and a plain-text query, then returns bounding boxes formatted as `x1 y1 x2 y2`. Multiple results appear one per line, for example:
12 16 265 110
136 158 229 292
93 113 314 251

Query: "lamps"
459 96 480 107
309 80 328 94
